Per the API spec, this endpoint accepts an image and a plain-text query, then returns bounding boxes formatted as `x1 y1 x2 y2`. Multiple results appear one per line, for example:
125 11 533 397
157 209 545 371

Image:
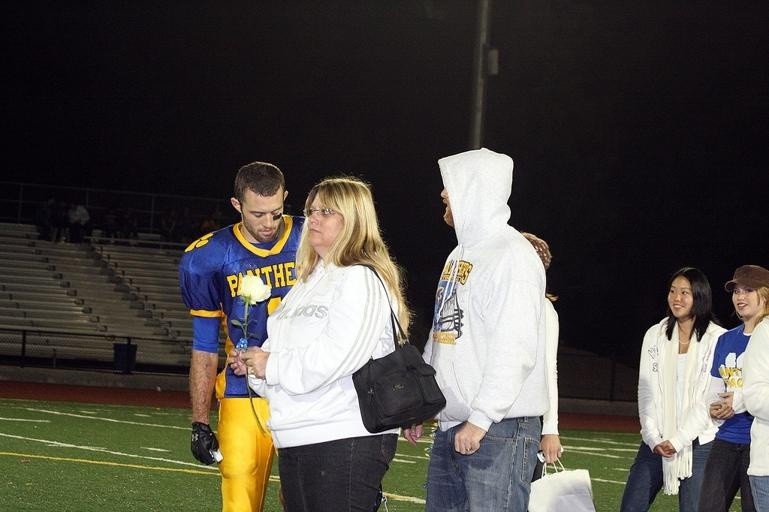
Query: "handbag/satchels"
527 457 596 512
352 344 447 434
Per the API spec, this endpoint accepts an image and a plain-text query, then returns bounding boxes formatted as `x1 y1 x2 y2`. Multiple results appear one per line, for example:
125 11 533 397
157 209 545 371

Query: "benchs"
0 218 220 367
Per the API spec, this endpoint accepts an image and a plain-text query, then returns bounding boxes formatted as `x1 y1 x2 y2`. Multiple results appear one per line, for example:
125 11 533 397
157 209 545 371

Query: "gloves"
191 421 219 466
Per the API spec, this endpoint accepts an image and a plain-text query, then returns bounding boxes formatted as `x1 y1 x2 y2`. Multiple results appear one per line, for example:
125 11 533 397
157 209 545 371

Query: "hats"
522 232 552 271
724 264 769 293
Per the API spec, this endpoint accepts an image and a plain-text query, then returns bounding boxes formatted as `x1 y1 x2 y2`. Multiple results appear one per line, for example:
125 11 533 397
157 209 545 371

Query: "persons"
179 161 306 512
519 232 562 483
403 147 550 512
228 176 416 512
35 199 95 243
622 265 769 512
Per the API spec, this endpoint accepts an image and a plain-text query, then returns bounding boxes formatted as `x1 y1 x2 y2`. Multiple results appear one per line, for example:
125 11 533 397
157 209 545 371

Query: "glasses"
306 208 340 217
